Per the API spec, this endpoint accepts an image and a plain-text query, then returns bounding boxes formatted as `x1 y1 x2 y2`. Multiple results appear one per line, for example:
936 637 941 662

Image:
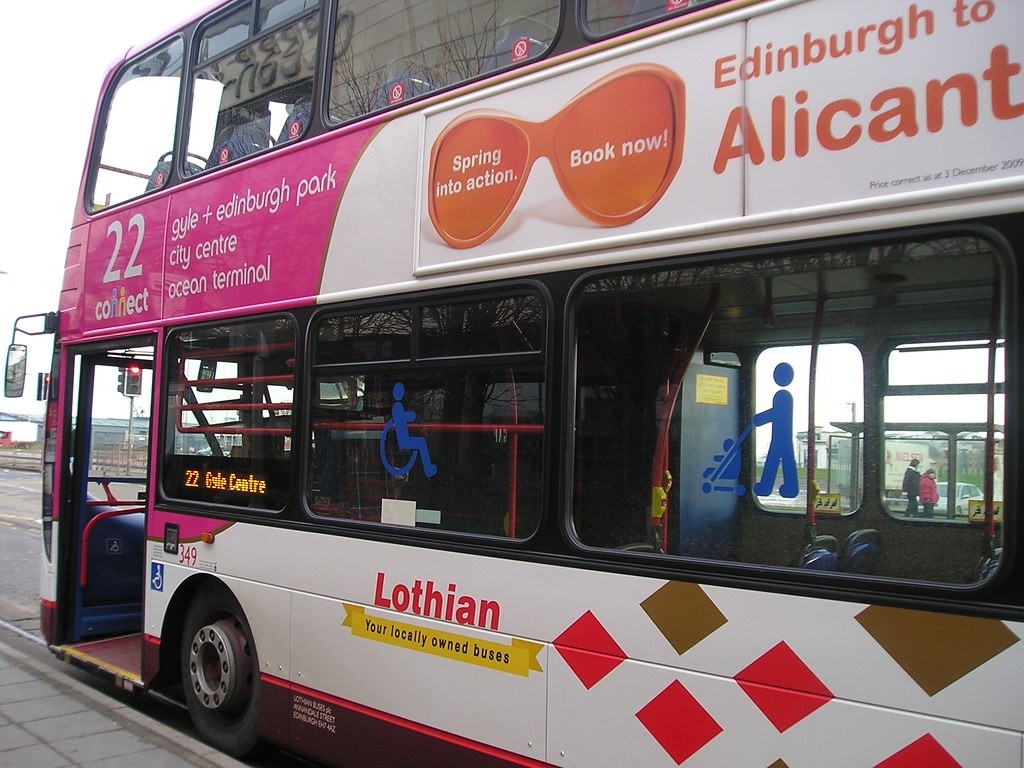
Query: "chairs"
203 126 277 171
275 94 355 145
842 529 904 581
376 58 447 110
796 534 841 574
145 151 208 193
482 14 557 74
614 0 706 29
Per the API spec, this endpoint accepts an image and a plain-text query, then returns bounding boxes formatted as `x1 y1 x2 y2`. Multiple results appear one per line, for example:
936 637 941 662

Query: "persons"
919 468 939 518
902 459 921 518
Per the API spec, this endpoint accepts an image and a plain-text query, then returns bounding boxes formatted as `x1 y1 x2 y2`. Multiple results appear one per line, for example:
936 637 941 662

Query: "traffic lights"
117 366 125 396
121 366 143 397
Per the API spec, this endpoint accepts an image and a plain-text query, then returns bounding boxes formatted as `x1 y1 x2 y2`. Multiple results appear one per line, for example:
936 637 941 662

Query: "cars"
915 481 983 517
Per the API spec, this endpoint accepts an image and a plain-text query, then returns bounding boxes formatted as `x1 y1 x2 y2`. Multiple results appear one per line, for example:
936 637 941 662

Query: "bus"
0 0 1023 767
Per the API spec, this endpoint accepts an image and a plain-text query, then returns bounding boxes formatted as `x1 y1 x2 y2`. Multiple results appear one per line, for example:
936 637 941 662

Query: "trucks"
835 438 940 502
